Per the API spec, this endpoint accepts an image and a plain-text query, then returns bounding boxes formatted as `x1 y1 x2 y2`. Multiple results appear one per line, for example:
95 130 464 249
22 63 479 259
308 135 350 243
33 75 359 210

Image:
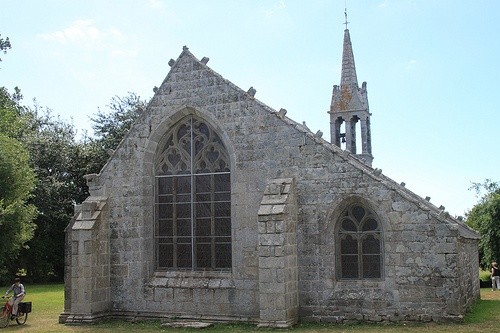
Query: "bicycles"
0 296 28 329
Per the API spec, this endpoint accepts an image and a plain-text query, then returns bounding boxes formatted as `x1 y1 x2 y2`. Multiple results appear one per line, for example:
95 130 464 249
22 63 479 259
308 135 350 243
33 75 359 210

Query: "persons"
490 262 500 291
2 278 26 321
